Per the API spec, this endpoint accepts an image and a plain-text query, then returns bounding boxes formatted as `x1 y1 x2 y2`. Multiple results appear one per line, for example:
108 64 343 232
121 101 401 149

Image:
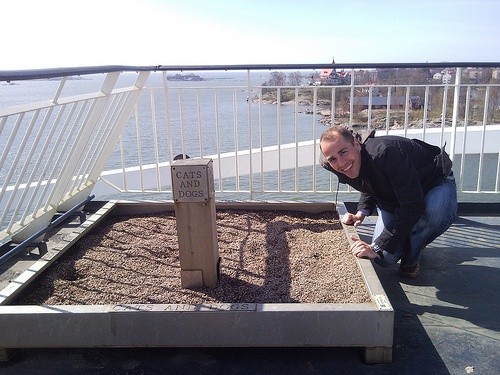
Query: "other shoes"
399 259 421 277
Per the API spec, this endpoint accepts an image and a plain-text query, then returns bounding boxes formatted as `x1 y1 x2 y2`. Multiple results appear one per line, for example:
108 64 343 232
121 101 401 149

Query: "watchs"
371 243 385 260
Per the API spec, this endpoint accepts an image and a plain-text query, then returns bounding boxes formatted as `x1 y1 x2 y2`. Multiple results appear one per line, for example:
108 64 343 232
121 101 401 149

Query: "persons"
319 125 458 278
